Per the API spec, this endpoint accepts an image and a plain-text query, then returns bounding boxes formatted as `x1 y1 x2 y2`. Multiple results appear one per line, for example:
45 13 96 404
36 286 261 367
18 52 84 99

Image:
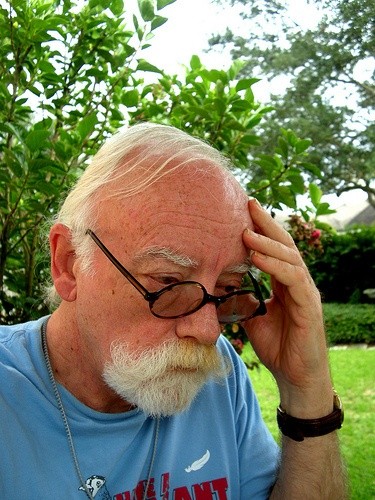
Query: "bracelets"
276 390 344 442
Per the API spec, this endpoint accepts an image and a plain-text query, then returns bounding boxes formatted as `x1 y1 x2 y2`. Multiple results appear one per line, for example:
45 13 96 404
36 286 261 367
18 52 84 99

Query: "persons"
0 126 351 500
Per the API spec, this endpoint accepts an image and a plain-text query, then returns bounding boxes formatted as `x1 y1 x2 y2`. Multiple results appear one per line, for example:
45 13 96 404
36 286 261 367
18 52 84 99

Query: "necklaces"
40 321 160 499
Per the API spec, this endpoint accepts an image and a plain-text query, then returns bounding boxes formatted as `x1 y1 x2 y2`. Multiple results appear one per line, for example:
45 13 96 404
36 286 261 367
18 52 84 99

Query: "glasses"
85 228 269 324
